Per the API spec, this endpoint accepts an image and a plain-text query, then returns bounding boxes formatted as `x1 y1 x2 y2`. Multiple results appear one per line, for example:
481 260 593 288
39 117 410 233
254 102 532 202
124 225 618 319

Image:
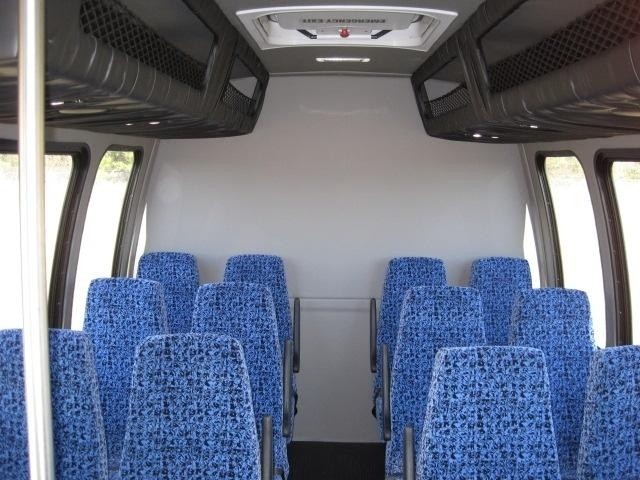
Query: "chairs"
0 252 301 479
368 256 639 478
